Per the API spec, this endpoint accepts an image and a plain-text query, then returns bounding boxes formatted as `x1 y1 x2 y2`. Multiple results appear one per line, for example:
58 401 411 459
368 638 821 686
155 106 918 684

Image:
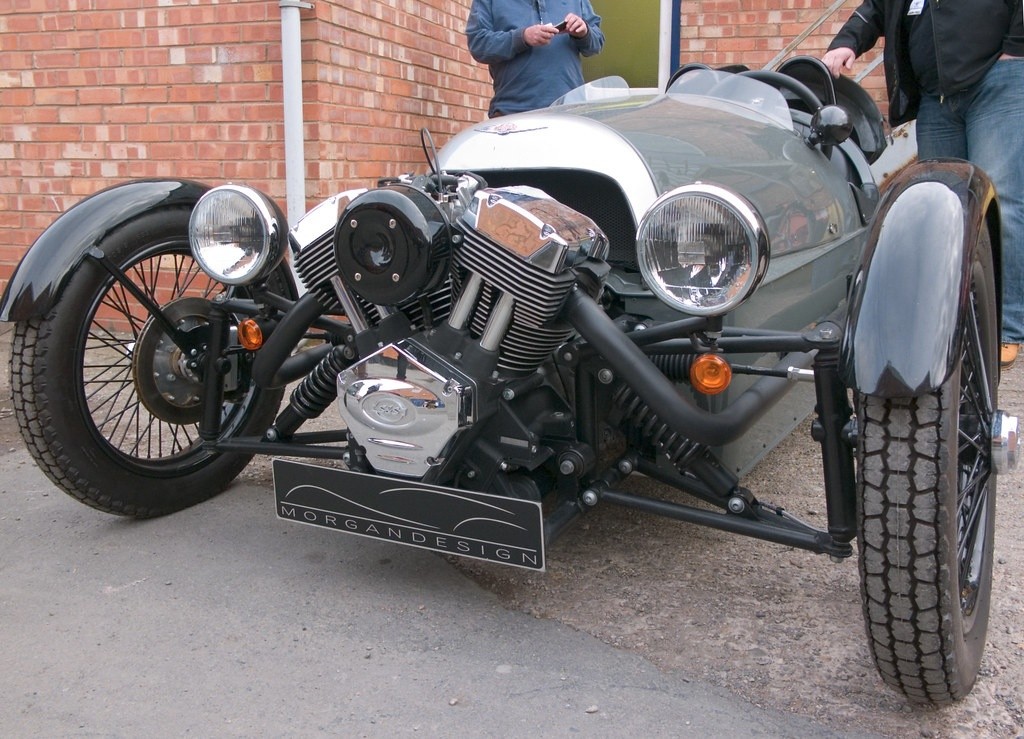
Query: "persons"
821 0 1024 369
464 0 605 118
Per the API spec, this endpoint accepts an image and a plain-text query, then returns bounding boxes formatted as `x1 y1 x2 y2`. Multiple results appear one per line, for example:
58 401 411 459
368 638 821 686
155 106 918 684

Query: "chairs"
773 55 837 116
665 60 721 96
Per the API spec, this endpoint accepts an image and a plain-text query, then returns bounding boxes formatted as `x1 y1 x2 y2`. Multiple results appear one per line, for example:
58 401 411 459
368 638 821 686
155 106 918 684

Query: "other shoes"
1001 342 1021 369
